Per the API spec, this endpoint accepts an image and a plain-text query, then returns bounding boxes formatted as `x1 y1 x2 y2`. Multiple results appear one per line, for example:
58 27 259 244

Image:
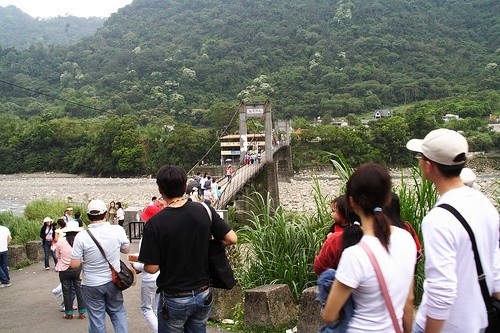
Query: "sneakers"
0 279 11 288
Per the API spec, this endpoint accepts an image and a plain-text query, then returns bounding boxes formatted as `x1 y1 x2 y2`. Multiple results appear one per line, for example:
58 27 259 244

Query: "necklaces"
167 198 186 207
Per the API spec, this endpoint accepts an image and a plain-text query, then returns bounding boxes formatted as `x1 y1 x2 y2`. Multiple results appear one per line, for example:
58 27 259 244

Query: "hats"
207 176 211 178
140 205 161 223
405 129 469 166
60 221 84 232
43 217 53 223
66 207 73 210
87 200 107 215
229 164 232 166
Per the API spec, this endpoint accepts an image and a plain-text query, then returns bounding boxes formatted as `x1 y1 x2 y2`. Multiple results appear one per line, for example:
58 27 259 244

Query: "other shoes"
79 313 86 319
46 266 50 270
72 304 78 310
63 314 73 319
59 303 65 312
54 266 57 272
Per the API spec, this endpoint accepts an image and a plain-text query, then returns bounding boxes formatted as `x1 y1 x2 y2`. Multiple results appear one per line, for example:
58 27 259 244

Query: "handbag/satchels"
110 260 134 290
483 295 500 333
218 261 221 262
195 199 235 291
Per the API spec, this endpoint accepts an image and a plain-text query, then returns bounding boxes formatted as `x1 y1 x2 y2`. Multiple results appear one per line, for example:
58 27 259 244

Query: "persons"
226 164 232 182
406 128 500 333
138 165 237 333
108 201 125 226
39 207 87 319
314 163 422 333
459 167 482 191
147 172 222 205
70 200 134 333
128 206 159 333
245 139 288 164
0 222 12 288
